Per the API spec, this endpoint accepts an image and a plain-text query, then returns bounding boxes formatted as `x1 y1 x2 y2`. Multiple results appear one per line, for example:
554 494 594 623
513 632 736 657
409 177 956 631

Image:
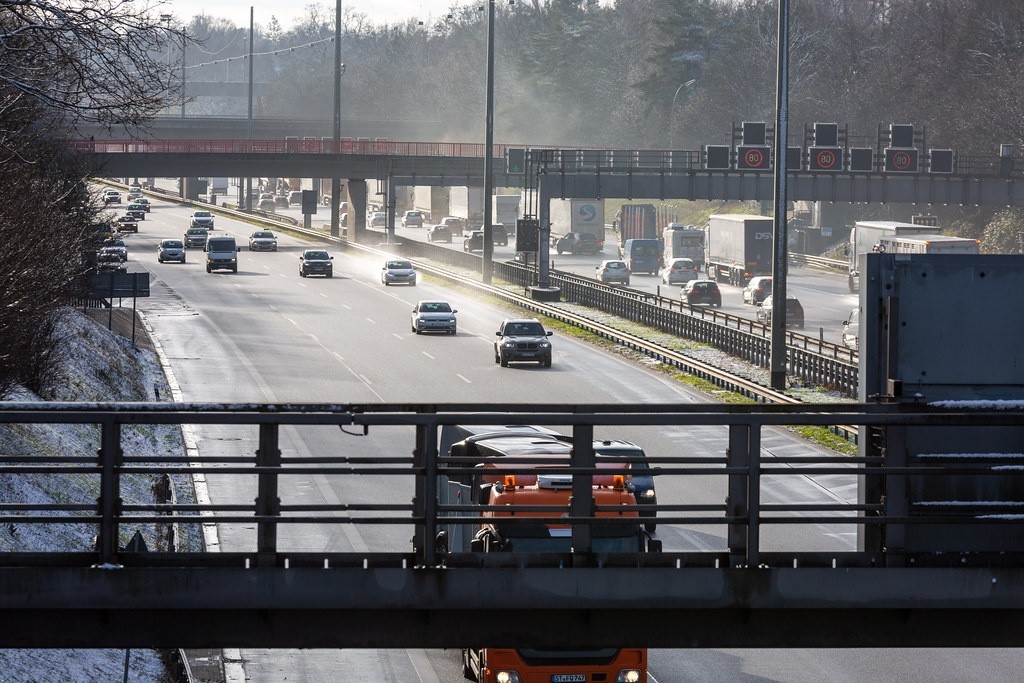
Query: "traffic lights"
507 147 526 175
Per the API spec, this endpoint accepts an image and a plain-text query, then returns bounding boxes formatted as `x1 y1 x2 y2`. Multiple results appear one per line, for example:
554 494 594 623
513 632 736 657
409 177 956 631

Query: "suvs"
494 318 553 368
742 276 804 327
595 260 630 285
299 249 334 278
438 217 508 253
401 210 422 228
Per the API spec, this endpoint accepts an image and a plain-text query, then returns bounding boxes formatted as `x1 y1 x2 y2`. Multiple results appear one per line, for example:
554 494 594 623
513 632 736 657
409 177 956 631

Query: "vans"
623 239 660 276
593 439 661 531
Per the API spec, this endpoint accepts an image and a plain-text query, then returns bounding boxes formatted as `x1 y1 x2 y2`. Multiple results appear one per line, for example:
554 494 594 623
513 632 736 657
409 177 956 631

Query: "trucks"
203 230 241 273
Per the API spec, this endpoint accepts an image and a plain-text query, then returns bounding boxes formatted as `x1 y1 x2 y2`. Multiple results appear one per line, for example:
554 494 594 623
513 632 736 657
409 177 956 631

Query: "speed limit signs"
736 145 771 171
884 148 919 173
809 147 844 172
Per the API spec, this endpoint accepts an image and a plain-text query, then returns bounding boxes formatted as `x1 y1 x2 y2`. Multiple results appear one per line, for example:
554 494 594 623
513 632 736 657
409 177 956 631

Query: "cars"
249 231 277 252
339 201 385 229
157 211 215 263
381 260 417 286
428 225 452 243
556 232 603 255
679 279 722 307
661 258 698 285
101 187 151 233
244 188 302 213
411 300 458 335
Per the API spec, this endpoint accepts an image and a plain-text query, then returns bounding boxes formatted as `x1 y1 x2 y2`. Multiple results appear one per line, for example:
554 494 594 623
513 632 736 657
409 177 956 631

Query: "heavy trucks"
410 186 521 236
515 189 773 287
843 220 980 305
436 425 663 683
